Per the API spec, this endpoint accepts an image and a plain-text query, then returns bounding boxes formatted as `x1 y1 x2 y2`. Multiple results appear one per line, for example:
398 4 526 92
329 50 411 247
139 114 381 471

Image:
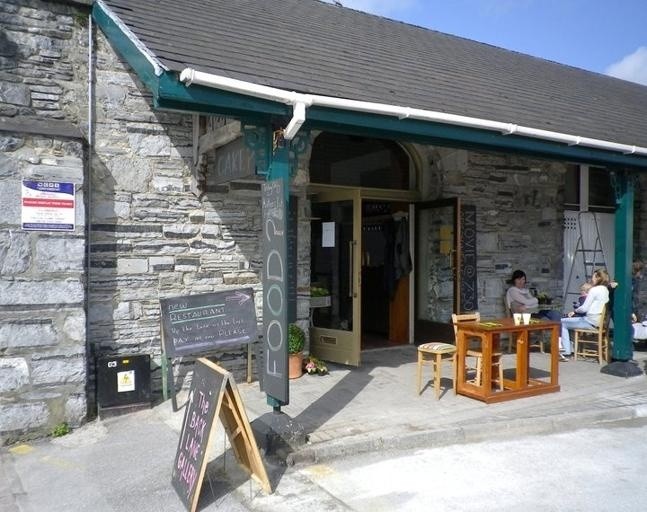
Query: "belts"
591 322 599 330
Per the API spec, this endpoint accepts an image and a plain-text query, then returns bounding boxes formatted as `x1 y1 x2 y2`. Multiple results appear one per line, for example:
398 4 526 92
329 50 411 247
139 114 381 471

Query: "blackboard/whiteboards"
160 287 260 358
460 204 478 313
214 135 256 186
171 360 224 512
260 176 289 404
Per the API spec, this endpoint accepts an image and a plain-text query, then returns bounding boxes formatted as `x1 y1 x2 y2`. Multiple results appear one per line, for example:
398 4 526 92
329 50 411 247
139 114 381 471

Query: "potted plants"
289 323 331 380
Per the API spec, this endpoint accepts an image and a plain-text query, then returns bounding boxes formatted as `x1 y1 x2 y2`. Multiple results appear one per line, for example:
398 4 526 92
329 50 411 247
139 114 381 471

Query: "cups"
513 313 531 326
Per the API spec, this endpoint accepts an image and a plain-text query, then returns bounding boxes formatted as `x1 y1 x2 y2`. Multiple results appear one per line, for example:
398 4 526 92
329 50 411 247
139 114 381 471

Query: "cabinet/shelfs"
389 276 410 344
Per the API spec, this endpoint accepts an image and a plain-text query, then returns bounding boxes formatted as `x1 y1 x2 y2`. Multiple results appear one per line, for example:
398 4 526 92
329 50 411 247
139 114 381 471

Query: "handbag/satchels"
385 245 410 280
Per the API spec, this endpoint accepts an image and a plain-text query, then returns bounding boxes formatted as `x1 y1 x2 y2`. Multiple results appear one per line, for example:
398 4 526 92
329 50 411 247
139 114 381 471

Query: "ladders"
560 211 608 317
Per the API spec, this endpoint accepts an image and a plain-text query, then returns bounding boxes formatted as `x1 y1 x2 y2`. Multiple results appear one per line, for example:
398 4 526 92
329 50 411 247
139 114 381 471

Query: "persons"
505 269 569 362
559 268 608 358
607 261 643 365
561 282 592 317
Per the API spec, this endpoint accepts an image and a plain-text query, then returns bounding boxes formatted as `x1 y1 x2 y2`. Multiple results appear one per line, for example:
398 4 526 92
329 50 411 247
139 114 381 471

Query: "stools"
417 342 458 400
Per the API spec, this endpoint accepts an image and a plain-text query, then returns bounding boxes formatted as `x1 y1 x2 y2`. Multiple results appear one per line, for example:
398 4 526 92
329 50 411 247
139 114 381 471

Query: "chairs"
450 297 612 404
